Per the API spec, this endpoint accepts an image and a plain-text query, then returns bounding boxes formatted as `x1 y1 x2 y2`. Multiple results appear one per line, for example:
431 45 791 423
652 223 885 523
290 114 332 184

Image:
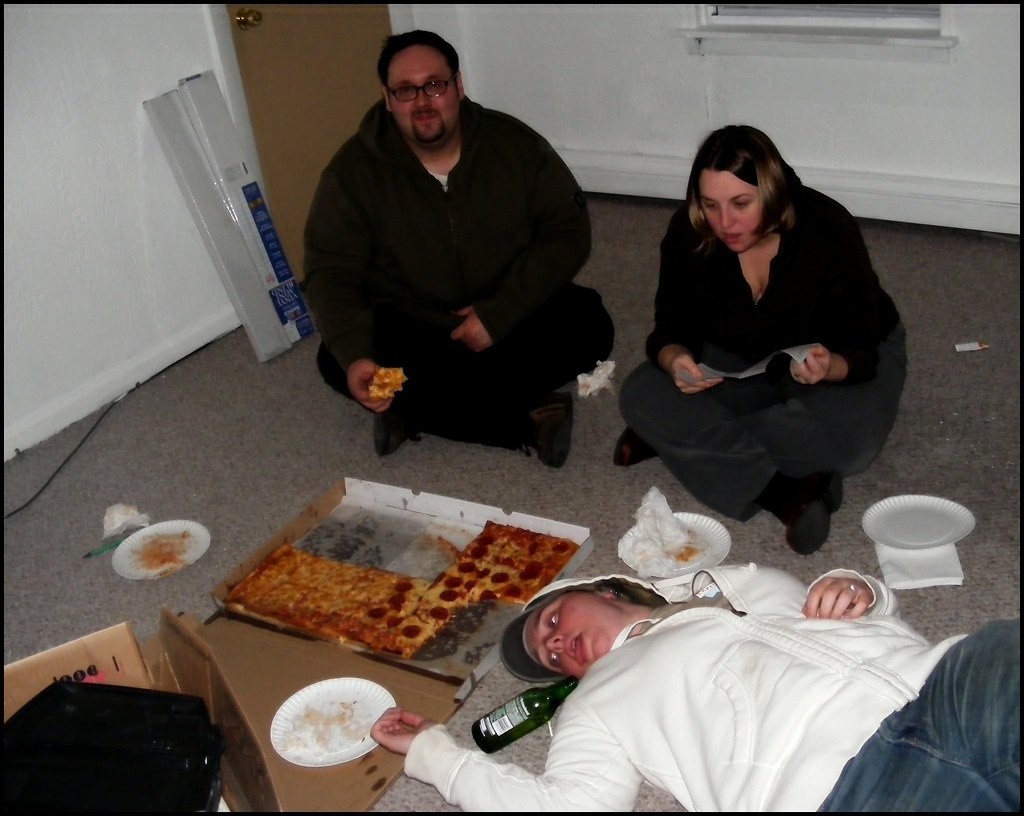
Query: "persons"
370 563 1022 811
614 125 906 555
300 28 614 468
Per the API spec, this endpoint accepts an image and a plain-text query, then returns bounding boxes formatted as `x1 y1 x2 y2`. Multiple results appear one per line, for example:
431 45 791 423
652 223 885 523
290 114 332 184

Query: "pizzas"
224 519 581 661
369 365 407 399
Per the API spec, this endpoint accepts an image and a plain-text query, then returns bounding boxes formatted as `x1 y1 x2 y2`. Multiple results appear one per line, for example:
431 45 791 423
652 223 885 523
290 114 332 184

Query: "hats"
499 587 570 682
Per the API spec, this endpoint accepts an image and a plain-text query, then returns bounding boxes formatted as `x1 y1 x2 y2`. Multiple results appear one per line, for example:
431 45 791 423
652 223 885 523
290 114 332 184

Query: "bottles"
471 675 581 754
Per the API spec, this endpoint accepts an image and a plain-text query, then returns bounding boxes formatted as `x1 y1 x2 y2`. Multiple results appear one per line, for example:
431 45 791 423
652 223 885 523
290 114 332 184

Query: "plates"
862 493 976 548
617 511 730 579
269 676 398 768
112 519 212 580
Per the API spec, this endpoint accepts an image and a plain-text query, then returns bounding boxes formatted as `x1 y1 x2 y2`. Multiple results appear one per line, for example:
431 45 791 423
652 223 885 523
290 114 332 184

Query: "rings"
847 584 857 593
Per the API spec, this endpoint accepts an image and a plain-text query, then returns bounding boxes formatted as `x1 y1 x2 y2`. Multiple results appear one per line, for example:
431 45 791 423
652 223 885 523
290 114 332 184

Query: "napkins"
876 540 963 590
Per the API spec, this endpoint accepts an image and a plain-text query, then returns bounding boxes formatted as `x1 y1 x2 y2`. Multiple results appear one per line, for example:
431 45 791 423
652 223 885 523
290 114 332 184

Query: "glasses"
386 73 457 103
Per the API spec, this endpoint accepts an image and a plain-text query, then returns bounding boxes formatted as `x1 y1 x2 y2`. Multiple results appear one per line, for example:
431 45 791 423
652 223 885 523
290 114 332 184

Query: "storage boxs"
7 478 595 814
144 89 293 363
177 68 315 344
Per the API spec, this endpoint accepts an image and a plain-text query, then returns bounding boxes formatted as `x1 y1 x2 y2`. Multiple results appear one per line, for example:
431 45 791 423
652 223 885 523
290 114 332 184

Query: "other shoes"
527 391 574 468
785 470 843 556
372 408 423 457
613 425 660 467
